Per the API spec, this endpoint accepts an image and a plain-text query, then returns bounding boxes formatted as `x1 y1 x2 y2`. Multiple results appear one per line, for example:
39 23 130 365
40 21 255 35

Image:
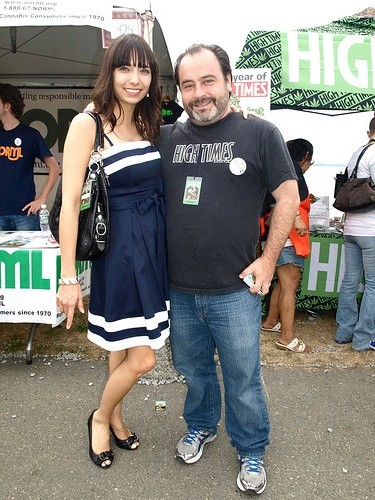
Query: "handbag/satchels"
48 110 110 261
332 144 375 212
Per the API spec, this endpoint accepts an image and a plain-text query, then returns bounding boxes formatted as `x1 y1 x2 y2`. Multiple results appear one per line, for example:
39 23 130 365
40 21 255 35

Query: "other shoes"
88 409 114 469
109 424 140 450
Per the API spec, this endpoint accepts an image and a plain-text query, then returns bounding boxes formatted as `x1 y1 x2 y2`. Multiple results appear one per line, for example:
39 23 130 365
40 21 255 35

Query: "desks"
259 227 365 317
0 229 94 365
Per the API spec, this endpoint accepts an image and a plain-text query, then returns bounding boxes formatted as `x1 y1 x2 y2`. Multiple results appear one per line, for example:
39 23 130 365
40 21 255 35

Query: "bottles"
39 204 49 231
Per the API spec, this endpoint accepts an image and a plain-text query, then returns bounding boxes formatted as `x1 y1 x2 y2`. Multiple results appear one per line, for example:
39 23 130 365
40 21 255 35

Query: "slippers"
261 322 281 332
275 338 306 353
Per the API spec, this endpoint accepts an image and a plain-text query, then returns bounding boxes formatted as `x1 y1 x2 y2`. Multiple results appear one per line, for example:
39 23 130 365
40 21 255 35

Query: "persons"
151 42 300 496
333 116 375 350
0 82 61 231
259 139 317 354
56 34 170 470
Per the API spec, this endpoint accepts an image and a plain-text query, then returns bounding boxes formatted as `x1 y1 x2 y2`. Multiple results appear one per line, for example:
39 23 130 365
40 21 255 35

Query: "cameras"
243 274 263 295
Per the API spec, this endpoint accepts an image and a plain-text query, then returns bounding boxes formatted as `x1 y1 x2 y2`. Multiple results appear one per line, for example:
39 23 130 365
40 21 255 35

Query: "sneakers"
237 453 267 494
174 430 217 463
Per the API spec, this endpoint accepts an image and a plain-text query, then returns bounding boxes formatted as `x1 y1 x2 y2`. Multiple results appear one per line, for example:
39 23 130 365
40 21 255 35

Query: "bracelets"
58 278 78 285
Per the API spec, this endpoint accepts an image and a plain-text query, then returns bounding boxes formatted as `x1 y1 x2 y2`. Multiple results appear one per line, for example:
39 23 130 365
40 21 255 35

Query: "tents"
0 0 177 217
234 5 375 116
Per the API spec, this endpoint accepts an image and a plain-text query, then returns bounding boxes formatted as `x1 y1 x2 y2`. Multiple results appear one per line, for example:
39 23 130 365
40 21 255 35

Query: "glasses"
309 159 314 165
